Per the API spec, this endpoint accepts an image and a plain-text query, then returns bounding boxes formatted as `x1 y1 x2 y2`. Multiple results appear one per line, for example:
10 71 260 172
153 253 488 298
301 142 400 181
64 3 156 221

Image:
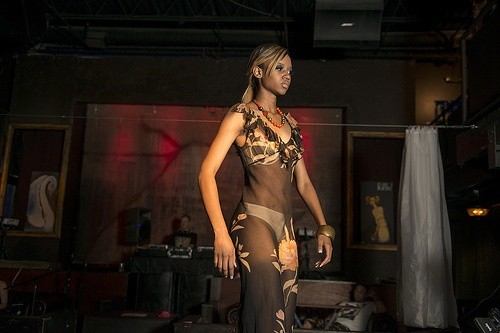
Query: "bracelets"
316 224 336 240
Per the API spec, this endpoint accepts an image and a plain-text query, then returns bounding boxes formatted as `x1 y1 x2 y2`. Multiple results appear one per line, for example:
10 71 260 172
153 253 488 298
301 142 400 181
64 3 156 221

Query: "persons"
323 280 386 331
197 41 336 333
169 214 197 248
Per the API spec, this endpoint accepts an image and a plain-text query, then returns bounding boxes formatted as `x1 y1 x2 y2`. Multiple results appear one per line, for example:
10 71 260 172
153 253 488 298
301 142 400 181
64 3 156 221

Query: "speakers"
125 208 152 244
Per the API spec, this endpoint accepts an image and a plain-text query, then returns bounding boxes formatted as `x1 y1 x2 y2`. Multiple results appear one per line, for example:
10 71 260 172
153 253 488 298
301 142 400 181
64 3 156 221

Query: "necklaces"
252 98 285 128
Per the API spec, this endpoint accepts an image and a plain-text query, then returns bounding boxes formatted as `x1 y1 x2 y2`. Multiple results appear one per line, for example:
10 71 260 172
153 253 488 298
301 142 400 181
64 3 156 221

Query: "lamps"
464 193 492 217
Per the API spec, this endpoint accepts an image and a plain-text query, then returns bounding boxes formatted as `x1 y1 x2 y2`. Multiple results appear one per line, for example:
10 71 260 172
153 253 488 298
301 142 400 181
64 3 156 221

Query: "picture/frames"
0 123 72 239
344 130 404 251
